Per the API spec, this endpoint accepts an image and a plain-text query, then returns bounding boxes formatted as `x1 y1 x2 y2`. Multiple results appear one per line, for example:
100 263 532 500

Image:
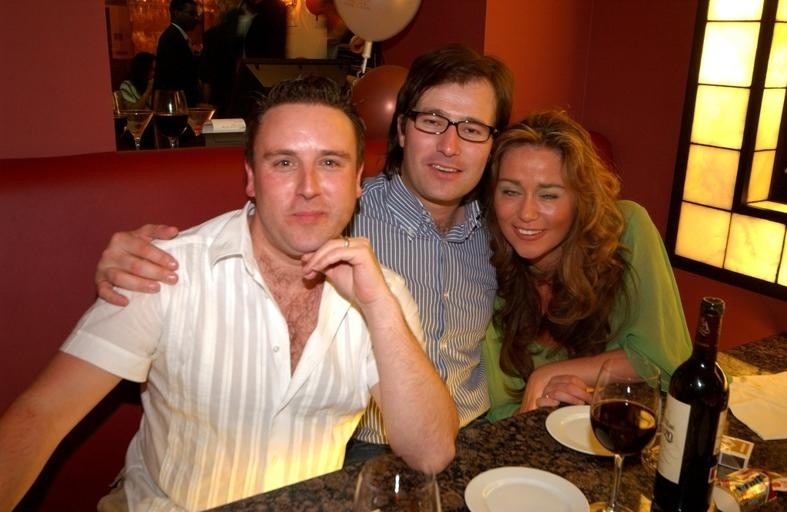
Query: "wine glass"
589 358 660 512
118 89 218 149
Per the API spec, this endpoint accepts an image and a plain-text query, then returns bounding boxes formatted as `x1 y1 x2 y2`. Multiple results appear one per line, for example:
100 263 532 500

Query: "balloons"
330 0 421 158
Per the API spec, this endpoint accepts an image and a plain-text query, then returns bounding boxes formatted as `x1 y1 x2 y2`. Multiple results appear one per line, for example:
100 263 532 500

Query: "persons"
482 111 695 424
96 43 515 472
0 74 460 512
117 0 264 152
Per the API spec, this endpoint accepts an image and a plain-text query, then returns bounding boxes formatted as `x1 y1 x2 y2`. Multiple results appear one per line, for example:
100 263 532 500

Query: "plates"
463 465 590 511
544 405 615 457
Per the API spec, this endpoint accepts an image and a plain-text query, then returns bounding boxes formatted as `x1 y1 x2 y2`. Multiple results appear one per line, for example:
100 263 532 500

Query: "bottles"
653 296 730 511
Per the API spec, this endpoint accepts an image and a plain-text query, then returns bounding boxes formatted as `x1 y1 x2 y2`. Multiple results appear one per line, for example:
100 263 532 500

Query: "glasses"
401 108 499 144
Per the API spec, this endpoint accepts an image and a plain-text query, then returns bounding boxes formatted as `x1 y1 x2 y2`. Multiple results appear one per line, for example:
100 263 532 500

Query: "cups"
353 454 440 511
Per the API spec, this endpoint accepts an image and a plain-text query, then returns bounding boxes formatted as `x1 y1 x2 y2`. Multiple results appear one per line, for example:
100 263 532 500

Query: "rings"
546 392 550 399
343 235 349 248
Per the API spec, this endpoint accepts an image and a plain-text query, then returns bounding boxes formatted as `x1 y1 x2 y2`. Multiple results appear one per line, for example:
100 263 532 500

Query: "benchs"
0 142 393 512
